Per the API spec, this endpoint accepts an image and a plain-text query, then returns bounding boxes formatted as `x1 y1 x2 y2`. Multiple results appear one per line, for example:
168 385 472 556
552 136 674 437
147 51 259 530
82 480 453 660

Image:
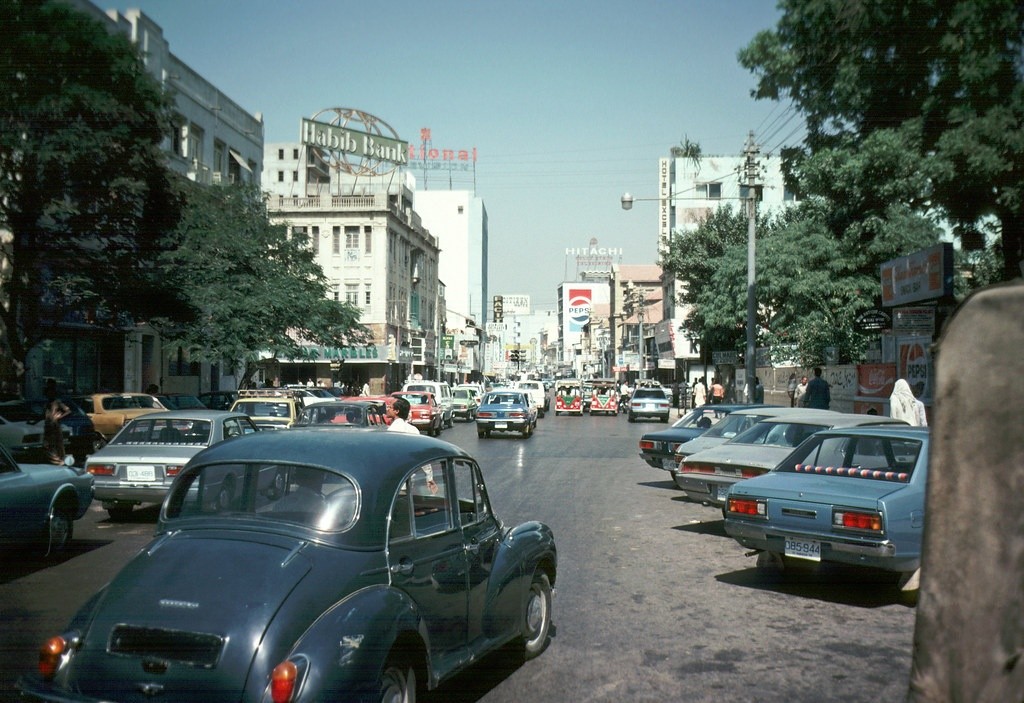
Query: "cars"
83 409 277 526
231 377 556 444
13 426 558 703
80 390 241 455
722 424 931 606
627 389 670 422
638 403 922 522
0 440 97 565
0 400 70 458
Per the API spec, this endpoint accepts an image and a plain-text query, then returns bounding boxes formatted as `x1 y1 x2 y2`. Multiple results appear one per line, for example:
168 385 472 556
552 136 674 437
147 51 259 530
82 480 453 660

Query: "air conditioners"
212 171 229 185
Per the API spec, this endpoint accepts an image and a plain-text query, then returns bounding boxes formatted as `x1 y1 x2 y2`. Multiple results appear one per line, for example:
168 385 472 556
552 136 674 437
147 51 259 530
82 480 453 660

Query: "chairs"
159 427 181 442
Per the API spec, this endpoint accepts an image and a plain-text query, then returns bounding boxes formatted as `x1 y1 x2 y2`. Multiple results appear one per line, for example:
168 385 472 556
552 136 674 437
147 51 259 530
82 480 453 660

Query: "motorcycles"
555 378 630 417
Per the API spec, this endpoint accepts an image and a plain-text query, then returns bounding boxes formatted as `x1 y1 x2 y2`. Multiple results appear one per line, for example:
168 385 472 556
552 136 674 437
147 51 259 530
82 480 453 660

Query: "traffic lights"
510 349 526 363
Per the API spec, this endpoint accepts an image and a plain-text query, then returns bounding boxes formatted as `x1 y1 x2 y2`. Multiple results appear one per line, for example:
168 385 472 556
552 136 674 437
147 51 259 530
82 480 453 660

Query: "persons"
145 384 159 396
889 378 928 428
40 398 72 467
443 378 480 387
787 367 831 410
361 380 370 397
615 376 764 419
386 396 439 496
241 377 325 389
